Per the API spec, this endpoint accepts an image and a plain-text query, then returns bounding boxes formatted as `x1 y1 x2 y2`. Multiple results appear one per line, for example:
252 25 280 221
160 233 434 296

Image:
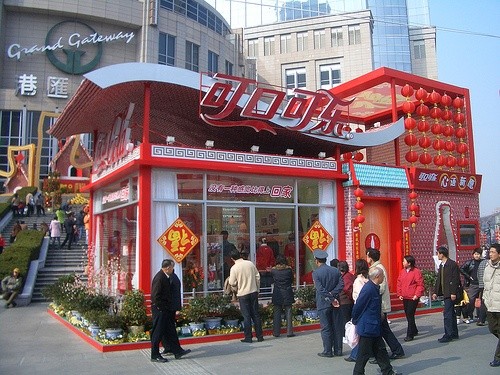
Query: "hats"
313 250 328 260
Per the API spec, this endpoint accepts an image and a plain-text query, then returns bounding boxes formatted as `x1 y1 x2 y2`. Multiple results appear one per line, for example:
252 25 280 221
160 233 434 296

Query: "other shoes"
4 305 9 309
258 339 264 342
404 337 414 341
438 336 453 343
318 353 333 357
390 353 405 359
476 321 485 326
414 333 418 336
241 340 252 343
332 351 342 356
463 319 469 324
392 372 403 375
273 334 280 337
470 319 473 322
369 360 378 364
160 350 174 356
287 334 296 337
456 318 459 324
12 303 17 308
489 360 500 367
344 357 356 362
452 336 459 339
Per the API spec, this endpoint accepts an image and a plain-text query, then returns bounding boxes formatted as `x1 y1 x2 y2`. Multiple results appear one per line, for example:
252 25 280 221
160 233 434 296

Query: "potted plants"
40 272 317 340
420 269 439 304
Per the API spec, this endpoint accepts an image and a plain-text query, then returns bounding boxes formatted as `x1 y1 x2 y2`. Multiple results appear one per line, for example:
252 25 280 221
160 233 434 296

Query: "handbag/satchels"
474 298 481 307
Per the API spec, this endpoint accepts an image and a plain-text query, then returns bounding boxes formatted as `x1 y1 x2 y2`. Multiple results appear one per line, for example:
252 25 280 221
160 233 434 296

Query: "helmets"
14 268 21 273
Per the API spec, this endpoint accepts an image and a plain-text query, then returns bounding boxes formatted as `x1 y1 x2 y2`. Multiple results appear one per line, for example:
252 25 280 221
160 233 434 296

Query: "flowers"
58 244 129 297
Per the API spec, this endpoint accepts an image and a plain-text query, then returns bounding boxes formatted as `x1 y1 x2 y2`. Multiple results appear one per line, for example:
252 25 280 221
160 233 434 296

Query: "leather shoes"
151 356 168 363
175 349 191 359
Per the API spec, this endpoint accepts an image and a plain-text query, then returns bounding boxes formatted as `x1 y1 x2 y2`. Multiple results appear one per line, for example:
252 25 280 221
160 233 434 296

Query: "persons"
285 233 306 292
229 250 264 343
151 260 191 363
61 213 77 249
0 233 4 255
432 246 464 342
160 259 181 356
344 259 379 364
220 228 282 300
459 248 486 324
339 261 356 337
0 267 24 309
477 249 491 325
397 255 424 341
49 216 62 249
311 249 345 358
10 191 89 247
266 257 295 337
482 243 500 367
108 230 121 270
366 247 405 365
330 258 340 267
454 280 470 325
351 266 403 375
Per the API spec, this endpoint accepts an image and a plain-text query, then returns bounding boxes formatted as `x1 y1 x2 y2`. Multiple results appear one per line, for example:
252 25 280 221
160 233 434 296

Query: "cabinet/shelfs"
186 235 224 289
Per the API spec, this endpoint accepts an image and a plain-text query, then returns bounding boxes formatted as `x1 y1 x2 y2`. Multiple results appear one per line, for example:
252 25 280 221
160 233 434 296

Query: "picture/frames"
257 213 279 234
